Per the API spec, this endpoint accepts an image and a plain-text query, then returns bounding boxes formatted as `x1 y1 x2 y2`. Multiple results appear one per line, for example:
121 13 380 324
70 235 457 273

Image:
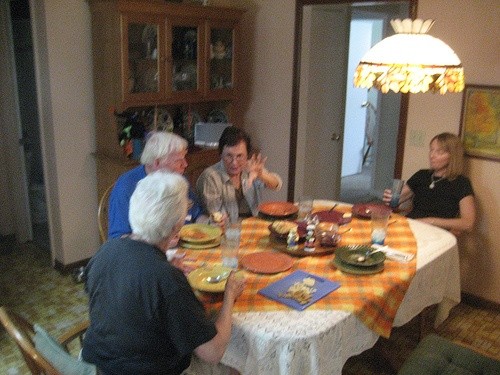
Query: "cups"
388 179 405 208
296 195 314 224
370 207 392 245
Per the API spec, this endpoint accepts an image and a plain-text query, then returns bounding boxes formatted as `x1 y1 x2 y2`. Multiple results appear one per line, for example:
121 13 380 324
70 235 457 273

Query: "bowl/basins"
267 221 304 238
180 222 223 242
314 223 342 246
335 245 386 267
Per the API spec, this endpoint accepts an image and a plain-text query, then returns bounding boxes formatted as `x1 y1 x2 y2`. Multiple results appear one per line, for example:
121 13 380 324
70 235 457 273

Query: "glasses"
223 152 248 163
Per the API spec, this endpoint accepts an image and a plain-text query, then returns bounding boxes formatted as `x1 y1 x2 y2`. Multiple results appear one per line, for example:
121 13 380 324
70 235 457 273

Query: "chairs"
0 306 97 375
397 333 500 375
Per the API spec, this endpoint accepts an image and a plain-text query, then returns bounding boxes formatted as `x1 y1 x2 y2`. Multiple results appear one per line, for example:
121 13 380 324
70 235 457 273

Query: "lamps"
352 0 465 93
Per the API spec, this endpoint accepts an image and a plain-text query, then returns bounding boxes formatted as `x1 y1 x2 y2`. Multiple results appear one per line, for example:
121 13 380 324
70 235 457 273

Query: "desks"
165 199 460 375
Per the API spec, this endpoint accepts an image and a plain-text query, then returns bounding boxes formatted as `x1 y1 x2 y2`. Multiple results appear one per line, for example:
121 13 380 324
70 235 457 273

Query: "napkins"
372 243 414 262
165 248 178 262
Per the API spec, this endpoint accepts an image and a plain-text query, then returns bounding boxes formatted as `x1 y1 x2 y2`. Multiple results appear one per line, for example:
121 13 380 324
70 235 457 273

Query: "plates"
179 241 220 249
258 201 299 217
351 203 392 218
333 253 385 275
208 108 229 123
241 251 293 274
188 265 247 293
311 211 353 225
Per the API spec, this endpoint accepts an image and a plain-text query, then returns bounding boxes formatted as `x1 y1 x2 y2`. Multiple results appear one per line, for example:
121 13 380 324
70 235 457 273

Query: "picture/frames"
458 84 500 163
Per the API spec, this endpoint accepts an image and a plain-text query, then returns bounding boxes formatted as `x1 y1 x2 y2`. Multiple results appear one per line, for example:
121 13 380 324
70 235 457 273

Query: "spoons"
207 271 230 283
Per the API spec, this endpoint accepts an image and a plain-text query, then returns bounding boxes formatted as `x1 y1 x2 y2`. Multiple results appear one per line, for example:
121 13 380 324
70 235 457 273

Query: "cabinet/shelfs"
84 0 249 243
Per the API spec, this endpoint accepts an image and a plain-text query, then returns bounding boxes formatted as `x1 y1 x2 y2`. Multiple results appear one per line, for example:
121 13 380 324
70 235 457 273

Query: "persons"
382 132 476 233
107 131 227 240
77 168 246 375
195 126 283 225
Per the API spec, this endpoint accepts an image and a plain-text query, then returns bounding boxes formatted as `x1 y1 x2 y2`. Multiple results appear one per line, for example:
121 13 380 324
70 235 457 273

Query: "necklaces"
429 173 447 189
